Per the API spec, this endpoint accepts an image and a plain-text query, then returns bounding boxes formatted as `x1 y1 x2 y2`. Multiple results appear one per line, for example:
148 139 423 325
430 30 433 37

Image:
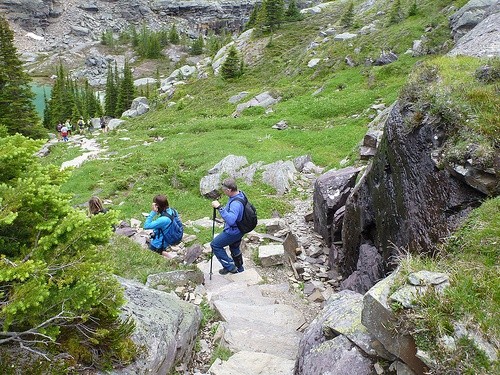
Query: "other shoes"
219 265 238 274
237 266 244 272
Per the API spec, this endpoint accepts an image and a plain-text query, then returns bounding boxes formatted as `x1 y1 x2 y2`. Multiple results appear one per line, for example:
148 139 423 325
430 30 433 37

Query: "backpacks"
229 190 257 234
159 209 184 246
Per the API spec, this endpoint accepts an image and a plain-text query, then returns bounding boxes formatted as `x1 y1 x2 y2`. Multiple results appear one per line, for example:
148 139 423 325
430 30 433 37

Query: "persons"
143 194 179 255
210 178 248 275
87 196 115 234
55 115 107 143
